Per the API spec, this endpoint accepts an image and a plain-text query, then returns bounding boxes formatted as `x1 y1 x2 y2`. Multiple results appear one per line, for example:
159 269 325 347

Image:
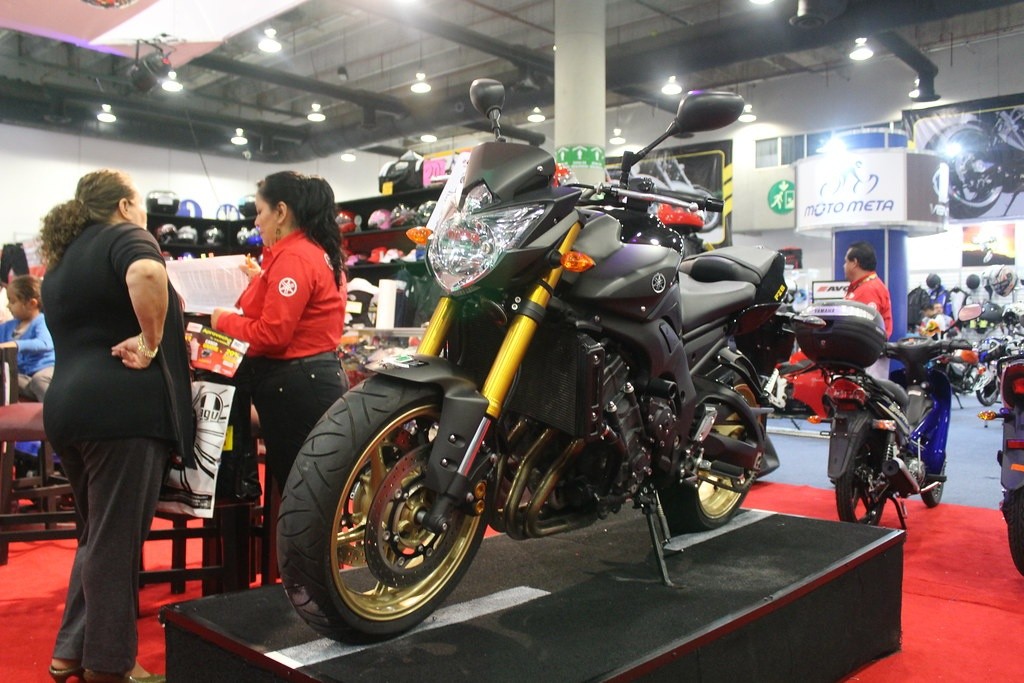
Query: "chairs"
0 334 279 597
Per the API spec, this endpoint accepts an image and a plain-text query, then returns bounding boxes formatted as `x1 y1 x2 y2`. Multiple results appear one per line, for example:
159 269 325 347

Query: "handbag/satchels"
156 356 262 519
379 150 425 194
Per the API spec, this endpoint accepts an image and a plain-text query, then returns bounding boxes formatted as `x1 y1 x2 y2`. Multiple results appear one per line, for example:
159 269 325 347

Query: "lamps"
339 123 357 163
410 39 432 93
230 103 248 145
737 62 757 123
306 93 326 121
609 94 626 144
527 105 545 122
660 74 683 94
258 20 282 52
162 55 183 92
95 77 117 123
849 36 874 61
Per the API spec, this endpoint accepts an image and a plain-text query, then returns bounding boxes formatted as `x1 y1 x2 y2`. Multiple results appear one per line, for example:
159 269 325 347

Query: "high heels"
50 665 166 683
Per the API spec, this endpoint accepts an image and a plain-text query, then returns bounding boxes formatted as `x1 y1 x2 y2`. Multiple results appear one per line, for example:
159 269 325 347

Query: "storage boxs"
796 300 886 368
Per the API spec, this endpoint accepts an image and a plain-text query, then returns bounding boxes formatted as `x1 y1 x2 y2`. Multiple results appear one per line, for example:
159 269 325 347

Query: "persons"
843 242 892 379
42 169 192 682
213 170 350 491
0 276 56 401
927 273 951 315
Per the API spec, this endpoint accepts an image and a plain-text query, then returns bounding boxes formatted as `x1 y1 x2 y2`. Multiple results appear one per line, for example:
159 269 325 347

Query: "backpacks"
345 271 447 328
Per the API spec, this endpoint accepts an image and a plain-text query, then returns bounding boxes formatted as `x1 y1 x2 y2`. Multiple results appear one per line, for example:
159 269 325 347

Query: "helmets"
177 225 197 245
966 274 980 289
368 200 437 230
335 211 356 233
981 265 1019 296
236 227 262 247
203 228 224 246
926 274 941 288
156 224 177 243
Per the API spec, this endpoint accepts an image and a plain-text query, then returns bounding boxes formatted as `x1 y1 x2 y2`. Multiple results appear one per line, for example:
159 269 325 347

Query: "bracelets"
138 331 158 358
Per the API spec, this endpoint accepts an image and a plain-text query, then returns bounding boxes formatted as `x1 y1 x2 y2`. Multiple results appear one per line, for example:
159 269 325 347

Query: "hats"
344 247 425 265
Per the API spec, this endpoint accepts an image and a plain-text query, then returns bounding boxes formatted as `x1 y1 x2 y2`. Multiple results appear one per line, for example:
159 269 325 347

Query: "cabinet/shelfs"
147 184 451 374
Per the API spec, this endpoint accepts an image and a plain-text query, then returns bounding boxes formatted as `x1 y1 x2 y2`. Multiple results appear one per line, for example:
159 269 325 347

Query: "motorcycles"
929 302 1024 576
795 297 972 527
276 77 796 646
